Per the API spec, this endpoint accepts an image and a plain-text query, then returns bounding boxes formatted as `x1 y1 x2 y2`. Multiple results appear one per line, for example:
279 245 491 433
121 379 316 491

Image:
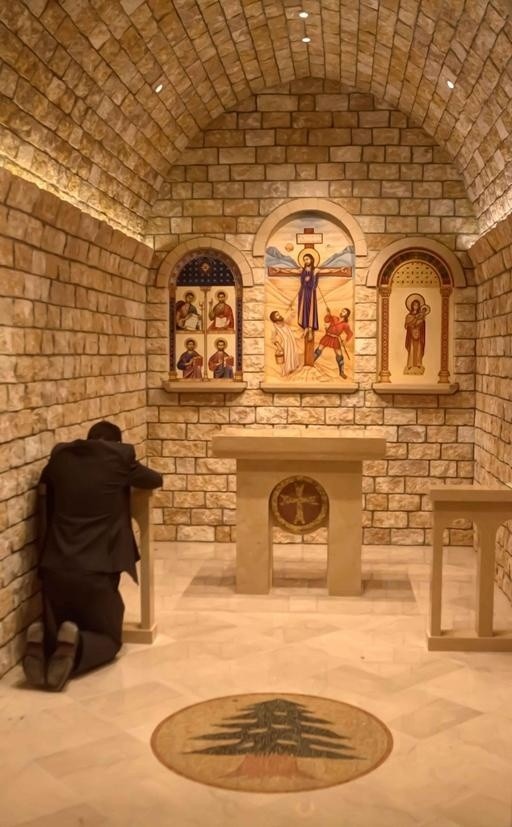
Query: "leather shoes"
20 618 81 693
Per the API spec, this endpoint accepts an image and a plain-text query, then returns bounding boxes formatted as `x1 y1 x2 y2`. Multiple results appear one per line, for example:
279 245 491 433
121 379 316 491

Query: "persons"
404 298 428 370
271 310 300 376
177 290 201 331
209 291 234 332
21 420 163 690
208 337 234 378
176 337 204 379
270 253 350 341
314 306 355 381
